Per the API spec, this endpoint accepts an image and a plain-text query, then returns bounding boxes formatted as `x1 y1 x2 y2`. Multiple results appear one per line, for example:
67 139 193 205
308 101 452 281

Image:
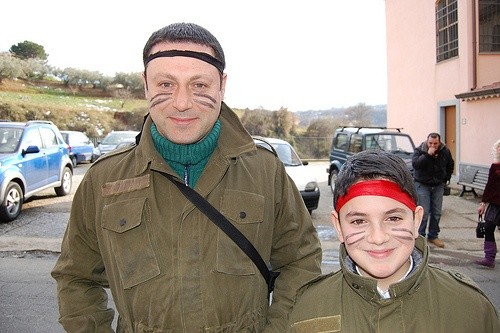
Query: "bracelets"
479 202 486 207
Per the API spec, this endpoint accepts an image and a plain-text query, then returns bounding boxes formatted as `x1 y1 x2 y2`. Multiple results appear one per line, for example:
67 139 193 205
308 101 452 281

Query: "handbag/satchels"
476 211 485 238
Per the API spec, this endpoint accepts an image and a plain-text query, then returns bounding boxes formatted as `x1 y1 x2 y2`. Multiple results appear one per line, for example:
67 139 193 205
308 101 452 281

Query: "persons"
474 140 500 268
413 133 454 247
288 151 500 333
52 21 322 333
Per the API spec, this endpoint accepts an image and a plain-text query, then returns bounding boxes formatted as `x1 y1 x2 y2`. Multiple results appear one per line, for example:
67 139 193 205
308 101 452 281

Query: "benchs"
457 169 490 199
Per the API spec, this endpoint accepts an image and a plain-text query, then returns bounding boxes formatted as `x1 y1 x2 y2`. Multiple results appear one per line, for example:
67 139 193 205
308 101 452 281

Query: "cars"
58 130 95 168
250 135 321 216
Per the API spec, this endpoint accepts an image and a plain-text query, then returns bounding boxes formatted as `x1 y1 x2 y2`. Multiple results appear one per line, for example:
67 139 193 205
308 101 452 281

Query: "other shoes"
475 262 496 269
427 238 444 248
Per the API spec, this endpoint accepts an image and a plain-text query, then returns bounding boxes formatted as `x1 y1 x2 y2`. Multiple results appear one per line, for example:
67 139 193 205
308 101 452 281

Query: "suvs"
0 119 74 220
325 125 418 195
92 129 142 162
